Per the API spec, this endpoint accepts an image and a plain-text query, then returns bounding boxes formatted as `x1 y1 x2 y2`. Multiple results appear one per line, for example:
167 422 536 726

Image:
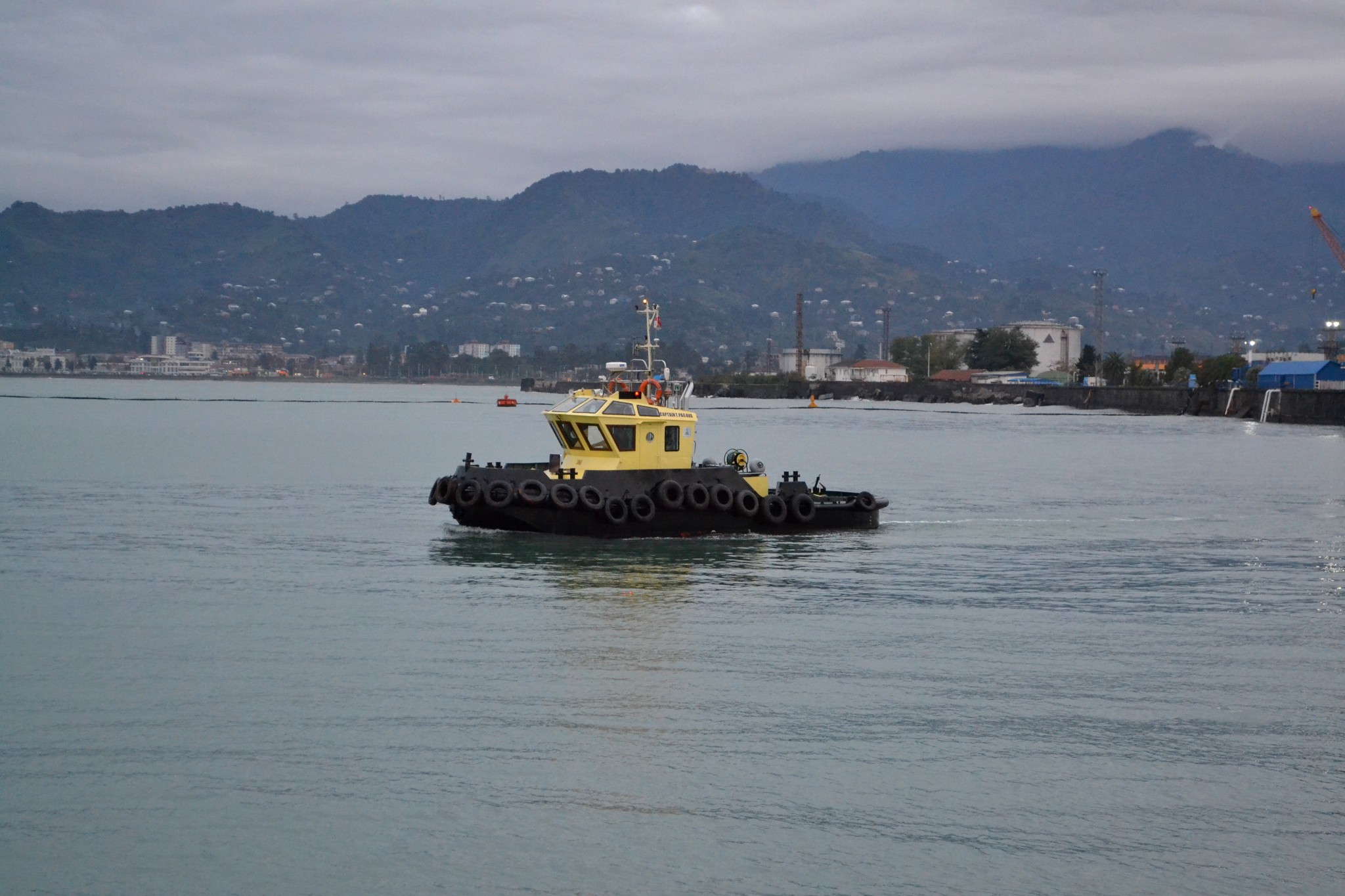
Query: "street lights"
1322 322 1345 358
1244 341 1255 354
1073 371 1076 383
927 342 932 377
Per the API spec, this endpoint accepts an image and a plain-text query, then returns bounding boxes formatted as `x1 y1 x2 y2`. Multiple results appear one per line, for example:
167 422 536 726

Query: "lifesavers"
428 477 442 505
434 476 459 506
607 377 626 395
789 493 816 523
629 493 655 522
638 378 663 405
856 491 877 512
655 479 685 510
517 478 547 506
550 483 579 510
483 480 514 510
455 478 482 508
579 485 605 512
708 483 734 512
602 495 629 525
760 494 787 525
734 489 759 518
684 483 710 510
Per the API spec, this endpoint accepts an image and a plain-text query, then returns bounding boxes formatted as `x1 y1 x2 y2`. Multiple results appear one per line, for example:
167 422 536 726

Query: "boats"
428 298 888 537
496 394 517 406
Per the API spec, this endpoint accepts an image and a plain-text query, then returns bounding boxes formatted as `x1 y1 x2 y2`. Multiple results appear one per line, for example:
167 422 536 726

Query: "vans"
488 376 495 380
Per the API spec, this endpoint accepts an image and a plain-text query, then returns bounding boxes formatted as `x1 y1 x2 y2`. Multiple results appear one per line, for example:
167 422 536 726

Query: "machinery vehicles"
1309 206 1345 301
